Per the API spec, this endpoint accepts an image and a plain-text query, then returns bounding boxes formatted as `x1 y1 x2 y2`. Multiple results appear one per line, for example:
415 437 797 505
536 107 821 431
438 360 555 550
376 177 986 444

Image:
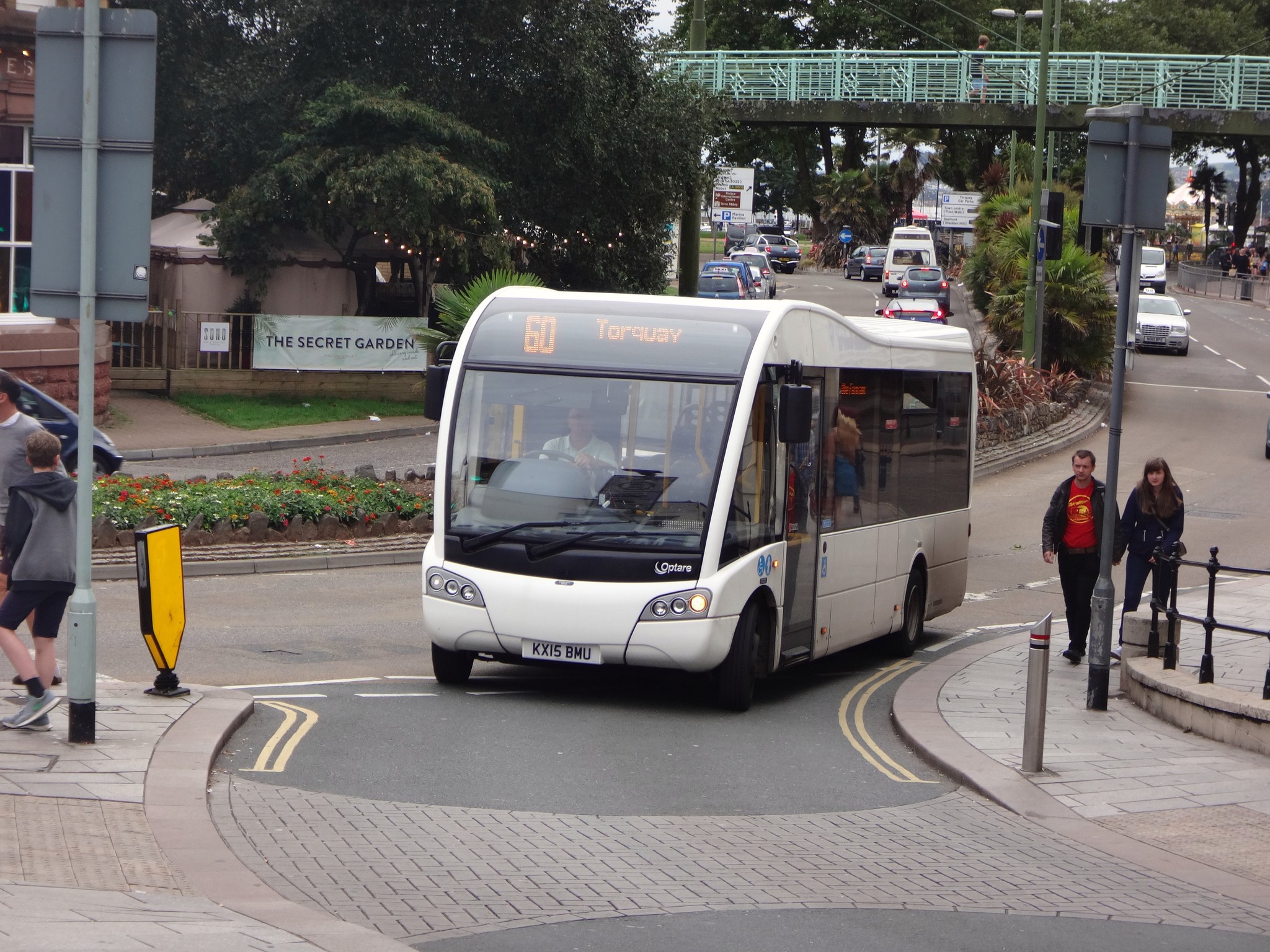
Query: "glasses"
567 412 592 421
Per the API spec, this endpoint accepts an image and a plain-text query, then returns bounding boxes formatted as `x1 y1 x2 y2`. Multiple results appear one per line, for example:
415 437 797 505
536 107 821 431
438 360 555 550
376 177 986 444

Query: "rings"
582 460 586 463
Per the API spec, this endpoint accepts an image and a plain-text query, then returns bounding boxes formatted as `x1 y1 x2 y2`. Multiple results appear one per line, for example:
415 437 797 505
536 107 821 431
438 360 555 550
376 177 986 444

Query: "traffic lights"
1216 203 1225 225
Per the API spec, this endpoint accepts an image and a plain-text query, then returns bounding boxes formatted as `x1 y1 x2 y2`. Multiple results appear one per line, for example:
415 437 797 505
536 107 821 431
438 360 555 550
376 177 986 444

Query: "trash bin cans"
1239 276 1254 301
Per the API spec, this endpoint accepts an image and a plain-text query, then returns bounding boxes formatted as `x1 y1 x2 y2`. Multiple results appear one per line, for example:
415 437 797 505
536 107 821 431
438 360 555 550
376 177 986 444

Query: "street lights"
991 8 1043 197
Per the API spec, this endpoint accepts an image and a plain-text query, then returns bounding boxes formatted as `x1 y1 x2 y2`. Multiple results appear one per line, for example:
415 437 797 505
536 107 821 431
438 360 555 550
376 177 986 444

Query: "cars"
1135 288 1192 356
697 271 754 299
749 266 772 300
875 296 954 326
729 251 780 297
897 265 954 310
702 261 760 299
700 222 725 232
844 245 888 282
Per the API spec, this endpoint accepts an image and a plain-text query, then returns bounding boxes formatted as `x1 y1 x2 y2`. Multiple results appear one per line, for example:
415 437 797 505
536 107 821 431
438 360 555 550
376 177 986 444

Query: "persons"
792 390 861 532
1186 240 1193 260
773 215 777 225
0 431 78 731
763 215 767 224
790 221 796 240
1042 449 1123 663
716 222 723 231
0 371 62 685
1111 457 1184 660
1159 233 1164 245
1167 233 1173 245
1221 244 1268 285
537 403 617 499
966 35 990 103
1172 240 1179 263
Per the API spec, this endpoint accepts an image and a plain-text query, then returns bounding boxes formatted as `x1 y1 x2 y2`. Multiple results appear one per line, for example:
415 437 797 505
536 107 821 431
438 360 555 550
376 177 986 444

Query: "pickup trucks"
725 234 801 274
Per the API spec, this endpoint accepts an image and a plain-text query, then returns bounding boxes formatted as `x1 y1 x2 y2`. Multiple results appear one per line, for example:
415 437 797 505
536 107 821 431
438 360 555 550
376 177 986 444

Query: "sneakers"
2 689 61 729
21 714 53 731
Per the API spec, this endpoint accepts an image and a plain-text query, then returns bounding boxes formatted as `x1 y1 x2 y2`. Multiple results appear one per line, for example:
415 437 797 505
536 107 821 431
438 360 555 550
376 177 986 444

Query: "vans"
16 378 123 475
881 225 937 297
1112 245 1171 294
724 220 795 255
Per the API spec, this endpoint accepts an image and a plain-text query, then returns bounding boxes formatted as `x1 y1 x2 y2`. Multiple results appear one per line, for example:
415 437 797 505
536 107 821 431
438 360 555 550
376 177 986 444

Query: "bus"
422 285 978 715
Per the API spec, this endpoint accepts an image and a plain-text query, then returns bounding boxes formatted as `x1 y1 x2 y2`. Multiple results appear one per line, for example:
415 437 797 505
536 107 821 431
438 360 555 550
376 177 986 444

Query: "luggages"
1239 276 1255 302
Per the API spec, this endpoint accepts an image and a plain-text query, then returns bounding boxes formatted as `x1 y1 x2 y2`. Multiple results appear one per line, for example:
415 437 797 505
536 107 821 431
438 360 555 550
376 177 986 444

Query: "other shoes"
13 670 62 686
1110 647 1122 659
1063 649 1086 662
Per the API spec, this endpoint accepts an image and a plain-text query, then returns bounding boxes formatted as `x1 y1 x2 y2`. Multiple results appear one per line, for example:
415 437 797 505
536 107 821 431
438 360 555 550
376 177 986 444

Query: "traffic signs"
711 166 754 223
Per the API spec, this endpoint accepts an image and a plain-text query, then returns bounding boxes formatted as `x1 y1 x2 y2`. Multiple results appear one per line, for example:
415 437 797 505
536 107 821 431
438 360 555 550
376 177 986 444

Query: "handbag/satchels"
1178 540 1187 557
1229 269 1237 277
